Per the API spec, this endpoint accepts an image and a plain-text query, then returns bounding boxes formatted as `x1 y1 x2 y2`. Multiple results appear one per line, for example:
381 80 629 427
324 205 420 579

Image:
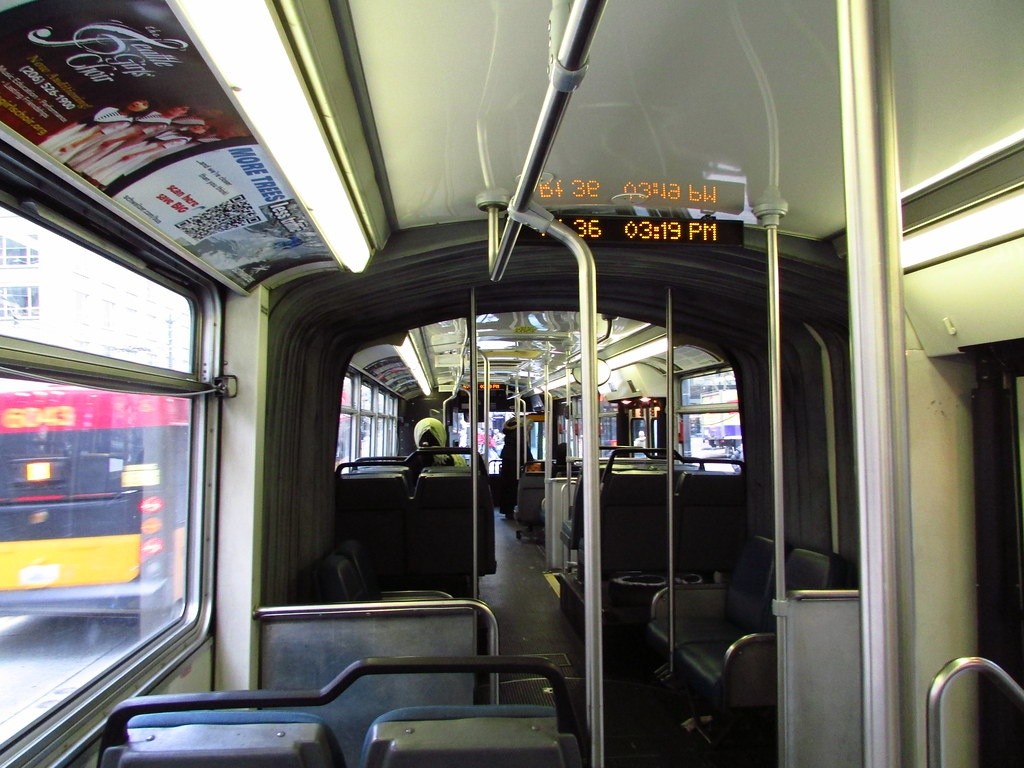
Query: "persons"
634 430 646 447
466 427 506 458
541 443 579 513
408 418 468 466
499 415 535 519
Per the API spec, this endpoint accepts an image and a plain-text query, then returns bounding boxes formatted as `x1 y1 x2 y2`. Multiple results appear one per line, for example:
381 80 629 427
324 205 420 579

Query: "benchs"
646 530 830 722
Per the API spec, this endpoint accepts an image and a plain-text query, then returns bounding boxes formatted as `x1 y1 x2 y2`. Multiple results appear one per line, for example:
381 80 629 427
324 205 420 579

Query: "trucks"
700 390 742 448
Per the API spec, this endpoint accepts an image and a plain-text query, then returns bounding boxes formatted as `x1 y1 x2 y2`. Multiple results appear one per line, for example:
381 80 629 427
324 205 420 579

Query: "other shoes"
505 511 514 520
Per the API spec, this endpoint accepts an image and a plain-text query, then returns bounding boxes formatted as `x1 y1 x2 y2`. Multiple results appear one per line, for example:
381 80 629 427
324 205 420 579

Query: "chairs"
99 445 852 768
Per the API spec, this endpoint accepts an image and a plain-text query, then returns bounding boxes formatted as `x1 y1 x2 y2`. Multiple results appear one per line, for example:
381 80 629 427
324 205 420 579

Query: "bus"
0 381 190 622
